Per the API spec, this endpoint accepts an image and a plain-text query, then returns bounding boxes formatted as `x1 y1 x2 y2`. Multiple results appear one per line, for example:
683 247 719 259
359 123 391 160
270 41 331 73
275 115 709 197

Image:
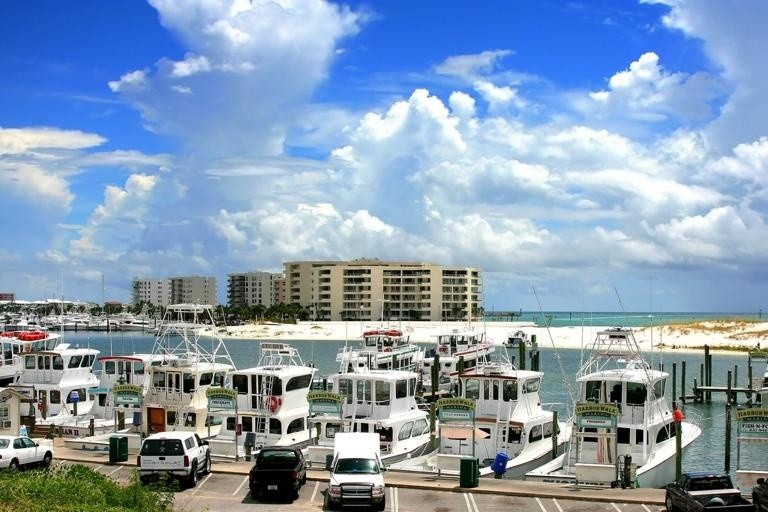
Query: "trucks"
323 431 388 512
246 445 308 504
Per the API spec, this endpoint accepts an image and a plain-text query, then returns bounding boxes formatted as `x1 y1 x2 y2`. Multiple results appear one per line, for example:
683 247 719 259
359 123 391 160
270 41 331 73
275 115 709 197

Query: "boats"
520 317 705 491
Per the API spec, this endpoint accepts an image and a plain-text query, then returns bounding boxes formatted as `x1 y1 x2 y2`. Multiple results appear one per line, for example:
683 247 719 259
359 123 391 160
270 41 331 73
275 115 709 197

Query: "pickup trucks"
661 468 754 512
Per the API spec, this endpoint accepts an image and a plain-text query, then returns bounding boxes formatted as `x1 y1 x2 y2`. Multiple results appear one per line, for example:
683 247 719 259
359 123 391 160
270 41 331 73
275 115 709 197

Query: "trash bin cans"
459 457 480 488
109 436 128 462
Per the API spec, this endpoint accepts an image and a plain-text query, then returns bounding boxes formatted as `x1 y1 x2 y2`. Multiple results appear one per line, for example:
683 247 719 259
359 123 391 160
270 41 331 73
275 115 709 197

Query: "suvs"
135 430 212 489
